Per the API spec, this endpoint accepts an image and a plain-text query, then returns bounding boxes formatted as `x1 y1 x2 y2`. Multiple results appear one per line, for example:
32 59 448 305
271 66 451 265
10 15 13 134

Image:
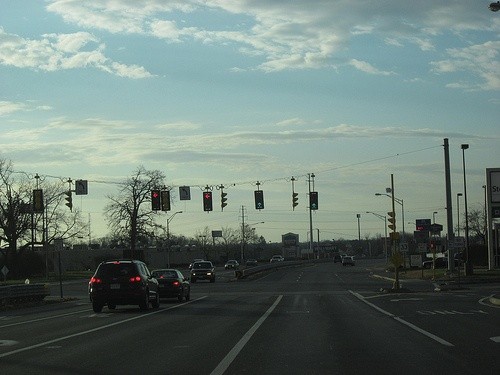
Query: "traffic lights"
65 191 72 210
151 190 160 210
203 192 212 211
310 192 318 210
389 232 400 240
221 191 227 208
255 190 264 209
292 192 298 208
388 212 396 230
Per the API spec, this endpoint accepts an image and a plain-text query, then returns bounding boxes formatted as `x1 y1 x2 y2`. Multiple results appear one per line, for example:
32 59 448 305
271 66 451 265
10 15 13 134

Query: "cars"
422 257 460 269
245 258 258 268
270 254 284 264
150 268 190 302
224 259 240 271
333 254 355 266
190 260 215 284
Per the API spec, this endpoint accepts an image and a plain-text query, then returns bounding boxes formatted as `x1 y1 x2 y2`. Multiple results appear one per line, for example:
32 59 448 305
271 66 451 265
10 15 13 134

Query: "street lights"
460 144 472 274
433 212 437 237
457 193 462 290
366 212 387 263
357 214 360 247
375 194 406 274
482 185 488 266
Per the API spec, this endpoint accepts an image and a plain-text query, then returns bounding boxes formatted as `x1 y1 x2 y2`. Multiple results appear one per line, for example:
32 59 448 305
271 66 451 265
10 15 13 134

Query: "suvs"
87 258 161 313
